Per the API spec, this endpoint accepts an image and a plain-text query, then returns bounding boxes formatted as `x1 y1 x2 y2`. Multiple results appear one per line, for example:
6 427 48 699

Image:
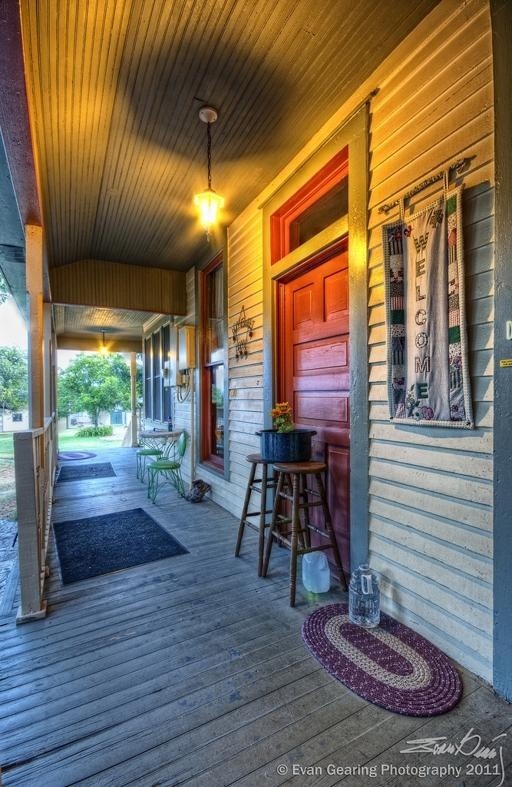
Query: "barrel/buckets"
347 562 380 628
301 550 330 595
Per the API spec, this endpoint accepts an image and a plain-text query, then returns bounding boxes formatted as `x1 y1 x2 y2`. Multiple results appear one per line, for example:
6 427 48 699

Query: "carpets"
50 507 193 590
55 449 120 485
301 600 462 718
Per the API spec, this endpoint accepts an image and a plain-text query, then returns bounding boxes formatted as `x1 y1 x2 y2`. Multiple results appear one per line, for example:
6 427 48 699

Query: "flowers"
263 400 301 434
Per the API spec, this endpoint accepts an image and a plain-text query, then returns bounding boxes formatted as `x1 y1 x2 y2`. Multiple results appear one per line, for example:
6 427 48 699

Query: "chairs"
134 427 191 504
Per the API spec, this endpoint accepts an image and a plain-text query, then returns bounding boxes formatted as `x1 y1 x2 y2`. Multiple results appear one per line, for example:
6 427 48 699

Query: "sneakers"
185 479 211 503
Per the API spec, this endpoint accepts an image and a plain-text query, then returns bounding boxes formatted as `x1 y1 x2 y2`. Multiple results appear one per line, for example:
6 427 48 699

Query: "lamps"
189 105 227 231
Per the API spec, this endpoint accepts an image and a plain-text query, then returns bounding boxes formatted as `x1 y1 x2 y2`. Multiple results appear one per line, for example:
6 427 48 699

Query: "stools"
235 451 351 609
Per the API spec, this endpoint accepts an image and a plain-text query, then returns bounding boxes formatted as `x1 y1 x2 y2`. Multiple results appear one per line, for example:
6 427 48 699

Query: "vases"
255 427 318 463
